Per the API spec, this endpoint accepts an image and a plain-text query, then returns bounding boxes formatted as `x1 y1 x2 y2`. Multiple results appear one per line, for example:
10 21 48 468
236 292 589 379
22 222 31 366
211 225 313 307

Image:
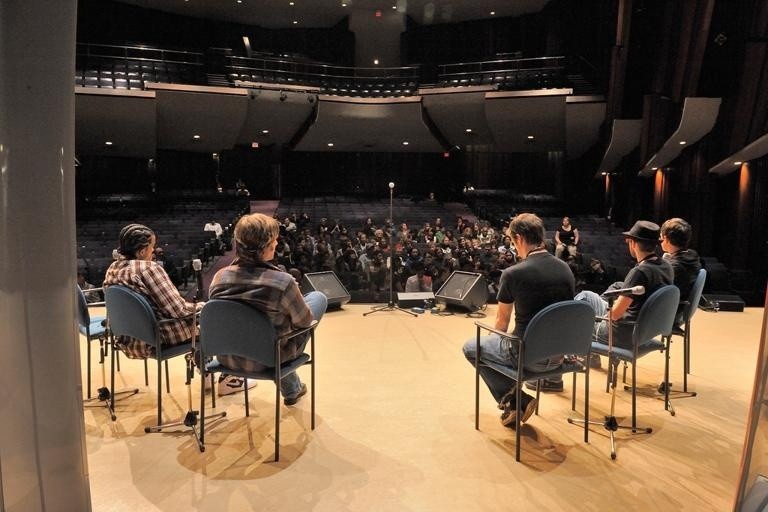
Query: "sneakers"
284 383 306 404
520 393 538 423
501 405 516 426
218 375 256 396
525 382 563 392
579 355 601 367
203 373 217 394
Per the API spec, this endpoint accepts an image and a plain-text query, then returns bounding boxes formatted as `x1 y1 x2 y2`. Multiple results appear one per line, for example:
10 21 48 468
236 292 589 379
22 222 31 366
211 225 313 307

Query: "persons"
463 182 474 194
102 223 258 396
583 216 701 369
236 184 251 196
462 212 577 426
207 213 328 406
76 271 101 304
154 247 178 278
554 216 580 260
269 209 523 293
204 217 224 241
525 218 674 393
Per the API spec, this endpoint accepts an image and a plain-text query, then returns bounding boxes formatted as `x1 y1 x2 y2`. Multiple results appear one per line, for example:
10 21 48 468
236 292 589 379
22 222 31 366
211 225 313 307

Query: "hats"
622 221 664 244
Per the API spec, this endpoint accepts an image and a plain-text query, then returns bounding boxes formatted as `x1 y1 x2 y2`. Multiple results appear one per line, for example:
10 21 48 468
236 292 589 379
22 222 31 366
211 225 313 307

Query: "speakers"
698 294 745 312
435 270 489 313
300 270 352 312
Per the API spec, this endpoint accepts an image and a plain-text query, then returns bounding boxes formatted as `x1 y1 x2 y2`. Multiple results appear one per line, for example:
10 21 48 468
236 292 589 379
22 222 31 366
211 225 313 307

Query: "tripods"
144 354 226 452
363 189 418 317
624 337 697 416
567 297 652 460
83 336 139 421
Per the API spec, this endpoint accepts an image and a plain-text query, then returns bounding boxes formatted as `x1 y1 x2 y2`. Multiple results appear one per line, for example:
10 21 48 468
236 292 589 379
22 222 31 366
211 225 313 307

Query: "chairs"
475 300 598 461
78 195 253 295
75 284 121 399
464 188 758 302
197 298 319 461
611 267 711 390
568 285 683 433
273 187 460 239
105 285 218 425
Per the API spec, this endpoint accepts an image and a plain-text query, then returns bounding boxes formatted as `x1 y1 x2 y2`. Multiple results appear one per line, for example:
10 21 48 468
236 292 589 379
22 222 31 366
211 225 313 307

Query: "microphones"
389 182 395 189
603 286 645 297
662 253 671 262
112 250 119 259
192 258 205 301
